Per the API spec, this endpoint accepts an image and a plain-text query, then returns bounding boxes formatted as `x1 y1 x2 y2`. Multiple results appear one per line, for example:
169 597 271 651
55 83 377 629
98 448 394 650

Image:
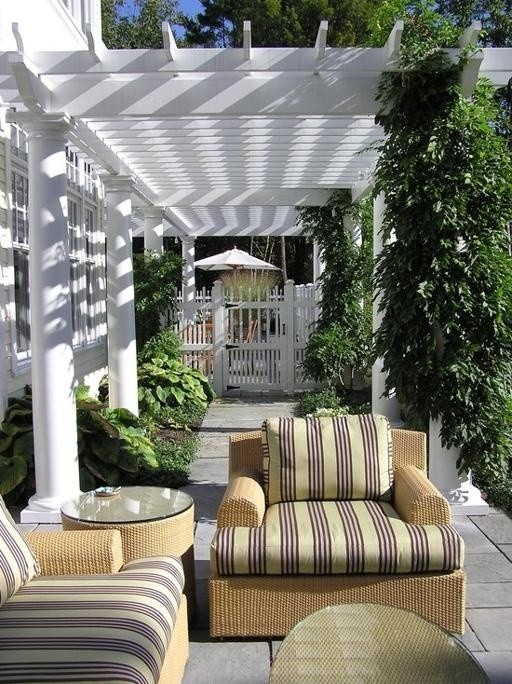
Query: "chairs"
204 427 469 642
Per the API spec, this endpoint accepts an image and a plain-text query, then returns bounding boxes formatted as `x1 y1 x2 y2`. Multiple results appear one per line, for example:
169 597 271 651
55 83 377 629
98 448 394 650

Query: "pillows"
261 411 394 504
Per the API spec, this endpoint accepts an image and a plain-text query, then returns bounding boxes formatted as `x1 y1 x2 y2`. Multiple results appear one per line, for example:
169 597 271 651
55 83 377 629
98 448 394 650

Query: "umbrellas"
192 245 283 300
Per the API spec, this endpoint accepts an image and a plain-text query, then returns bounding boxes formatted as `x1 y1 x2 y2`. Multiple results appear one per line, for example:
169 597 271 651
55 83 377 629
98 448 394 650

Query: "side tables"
48 484 198 627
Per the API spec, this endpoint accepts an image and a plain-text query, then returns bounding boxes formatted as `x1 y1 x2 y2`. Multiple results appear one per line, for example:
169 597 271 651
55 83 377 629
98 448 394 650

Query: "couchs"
0 495 192 684
